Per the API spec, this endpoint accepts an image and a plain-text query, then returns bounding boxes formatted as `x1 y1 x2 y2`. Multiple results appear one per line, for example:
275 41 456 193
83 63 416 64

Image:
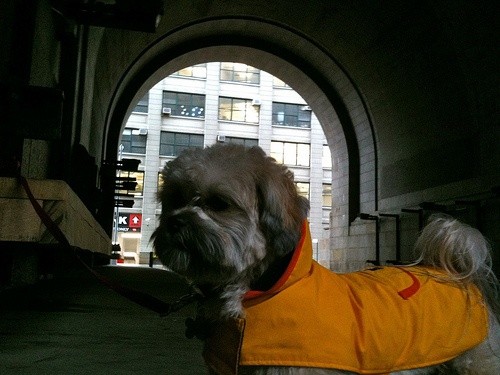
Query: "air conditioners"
218 135 225 141
162 108 171 114
252 98 262 105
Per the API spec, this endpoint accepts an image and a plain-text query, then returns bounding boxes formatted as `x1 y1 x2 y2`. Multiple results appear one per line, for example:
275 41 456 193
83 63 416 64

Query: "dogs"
149 141 500 375
